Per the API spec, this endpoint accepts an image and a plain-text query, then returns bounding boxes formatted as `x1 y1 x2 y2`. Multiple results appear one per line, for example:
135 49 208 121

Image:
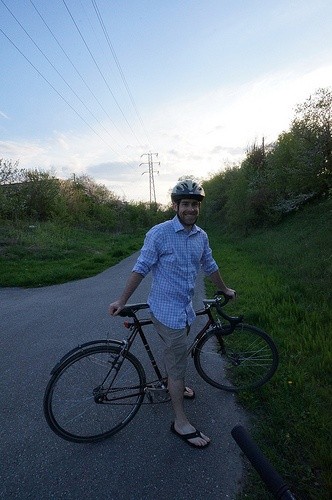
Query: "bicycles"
42 289 278 442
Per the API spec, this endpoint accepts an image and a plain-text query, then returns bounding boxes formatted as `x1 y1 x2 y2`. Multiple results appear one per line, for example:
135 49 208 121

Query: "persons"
107 178 237 450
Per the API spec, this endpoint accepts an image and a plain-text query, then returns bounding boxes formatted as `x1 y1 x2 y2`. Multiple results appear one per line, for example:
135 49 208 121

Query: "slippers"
183 387 195 399
171 420 210 449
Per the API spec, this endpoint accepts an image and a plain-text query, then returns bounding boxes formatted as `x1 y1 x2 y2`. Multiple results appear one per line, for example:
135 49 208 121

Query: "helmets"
171 180 205 201
